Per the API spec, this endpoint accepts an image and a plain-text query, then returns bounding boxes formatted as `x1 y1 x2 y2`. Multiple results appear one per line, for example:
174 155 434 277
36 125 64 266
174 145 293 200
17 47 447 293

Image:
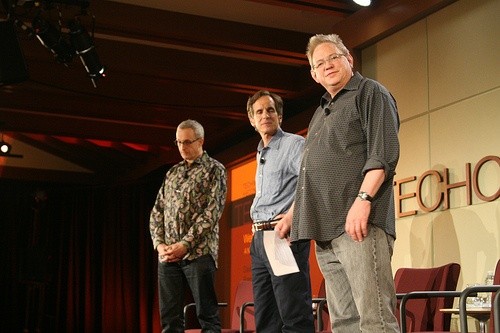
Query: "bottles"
485 271 494 305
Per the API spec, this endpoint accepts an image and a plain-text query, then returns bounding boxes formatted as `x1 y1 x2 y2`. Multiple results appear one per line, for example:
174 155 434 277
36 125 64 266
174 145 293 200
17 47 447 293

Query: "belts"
254 221 277 231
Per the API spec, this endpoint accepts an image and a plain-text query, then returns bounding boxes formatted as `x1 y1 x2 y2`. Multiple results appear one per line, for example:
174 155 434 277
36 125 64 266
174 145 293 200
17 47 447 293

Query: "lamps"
0 0 110 87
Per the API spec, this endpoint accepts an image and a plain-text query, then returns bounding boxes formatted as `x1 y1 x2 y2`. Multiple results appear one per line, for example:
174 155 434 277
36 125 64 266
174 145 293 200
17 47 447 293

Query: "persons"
247 90 311 333
149 120 227 333
289 34 402 333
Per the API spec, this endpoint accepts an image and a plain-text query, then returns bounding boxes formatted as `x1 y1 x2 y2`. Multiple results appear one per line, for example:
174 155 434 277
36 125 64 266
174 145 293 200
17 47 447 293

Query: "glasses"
312 53 345 70
175 137 202 145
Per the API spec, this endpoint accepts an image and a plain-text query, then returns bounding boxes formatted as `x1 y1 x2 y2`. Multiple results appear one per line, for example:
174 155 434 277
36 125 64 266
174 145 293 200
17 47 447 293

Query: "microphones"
323 108 330 116
182 173 189 179
259 158 265 165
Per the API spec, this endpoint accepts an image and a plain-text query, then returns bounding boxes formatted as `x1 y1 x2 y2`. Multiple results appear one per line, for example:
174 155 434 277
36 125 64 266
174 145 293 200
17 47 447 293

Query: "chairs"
184 281 255 333
312 259 500 333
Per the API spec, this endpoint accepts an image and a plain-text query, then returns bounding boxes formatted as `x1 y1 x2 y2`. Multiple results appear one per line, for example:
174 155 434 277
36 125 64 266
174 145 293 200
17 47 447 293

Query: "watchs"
358 192 373 202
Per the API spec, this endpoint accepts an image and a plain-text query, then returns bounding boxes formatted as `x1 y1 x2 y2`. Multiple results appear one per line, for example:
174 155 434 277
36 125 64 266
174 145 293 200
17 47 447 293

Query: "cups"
465 281 487 307
